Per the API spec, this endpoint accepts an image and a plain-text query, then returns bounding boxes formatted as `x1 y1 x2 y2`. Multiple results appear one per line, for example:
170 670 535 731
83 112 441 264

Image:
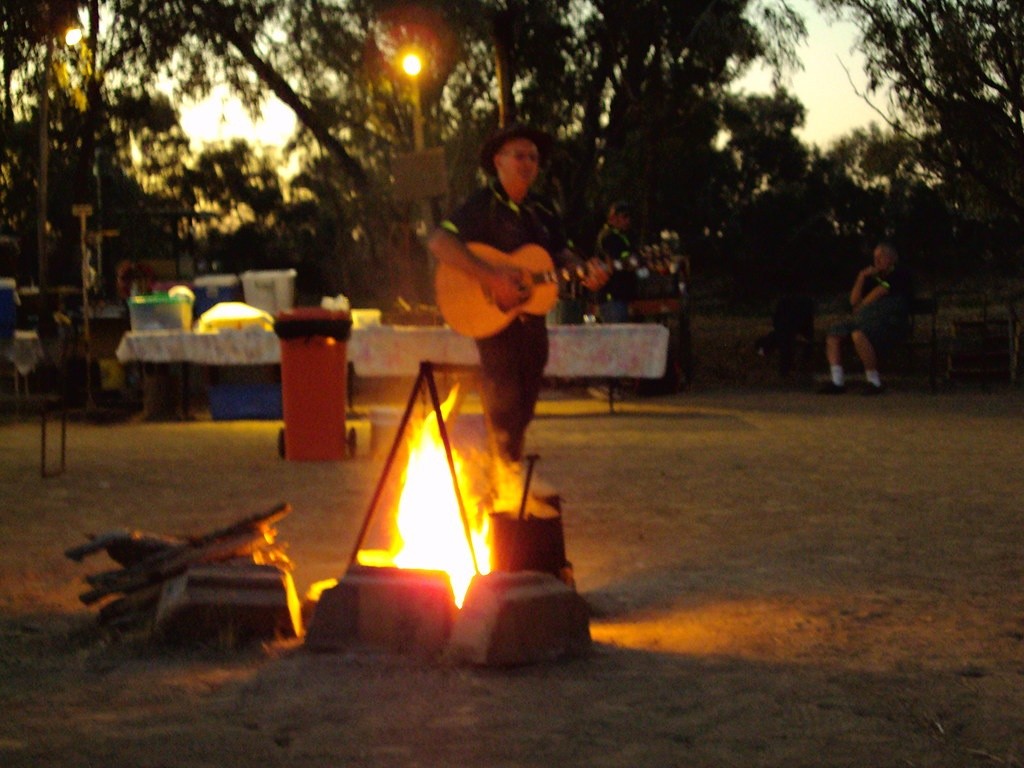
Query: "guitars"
432 234 691 343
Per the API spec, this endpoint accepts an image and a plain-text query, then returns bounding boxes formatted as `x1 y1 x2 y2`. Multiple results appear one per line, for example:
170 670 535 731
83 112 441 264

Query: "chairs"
886 298 938 377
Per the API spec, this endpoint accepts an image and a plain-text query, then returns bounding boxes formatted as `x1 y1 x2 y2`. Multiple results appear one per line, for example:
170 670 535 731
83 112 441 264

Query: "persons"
426 124 609 471
588 203 639 400
818 243 910 396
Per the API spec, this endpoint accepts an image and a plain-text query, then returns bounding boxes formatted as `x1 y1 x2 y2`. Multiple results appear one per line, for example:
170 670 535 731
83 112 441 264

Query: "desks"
347 323 670 412
116 328 376 421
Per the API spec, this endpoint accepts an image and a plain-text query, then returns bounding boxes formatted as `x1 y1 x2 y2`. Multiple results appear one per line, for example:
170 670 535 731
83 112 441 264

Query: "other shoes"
587 385 620 400
815 381 846 395
855 382 885 395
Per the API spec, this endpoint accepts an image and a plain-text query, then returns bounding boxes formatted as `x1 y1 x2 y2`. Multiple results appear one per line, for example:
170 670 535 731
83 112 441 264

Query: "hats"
480 124 552 175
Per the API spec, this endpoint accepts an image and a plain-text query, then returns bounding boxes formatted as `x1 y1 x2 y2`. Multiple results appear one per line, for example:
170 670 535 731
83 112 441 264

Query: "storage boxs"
1 269 297 420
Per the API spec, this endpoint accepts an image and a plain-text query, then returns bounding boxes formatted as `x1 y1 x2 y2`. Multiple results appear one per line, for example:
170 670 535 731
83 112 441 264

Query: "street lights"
32 22 79 286
400 52 423 231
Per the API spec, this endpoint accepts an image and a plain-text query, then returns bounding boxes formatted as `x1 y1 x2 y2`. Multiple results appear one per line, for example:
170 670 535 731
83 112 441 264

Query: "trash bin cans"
269 305 358 463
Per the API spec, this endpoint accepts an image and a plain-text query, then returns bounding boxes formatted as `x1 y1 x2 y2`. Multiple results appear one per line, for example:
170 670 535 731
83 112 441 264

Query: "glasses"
499 151 537 161
